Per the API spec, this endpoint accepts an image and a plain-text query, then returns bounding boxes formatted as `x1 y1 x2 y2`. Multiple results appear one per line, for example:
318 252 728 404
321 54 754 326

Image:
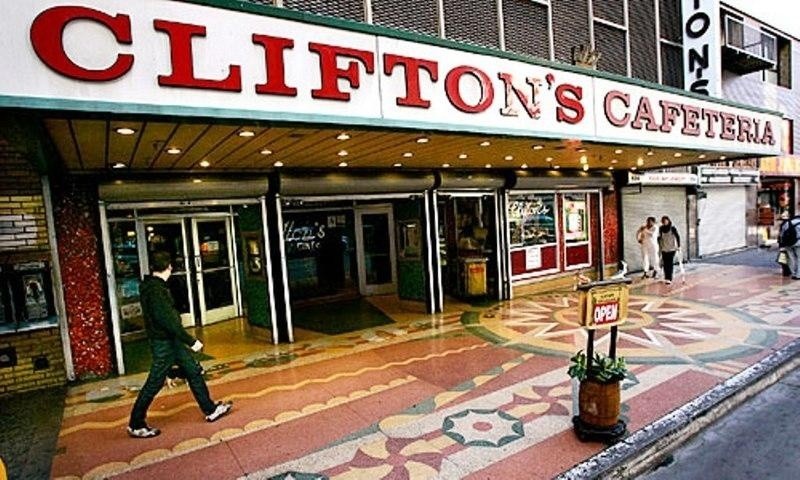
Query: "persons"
780 215 800 279
126 252 234 439
655 216 681 286
636 215 662 279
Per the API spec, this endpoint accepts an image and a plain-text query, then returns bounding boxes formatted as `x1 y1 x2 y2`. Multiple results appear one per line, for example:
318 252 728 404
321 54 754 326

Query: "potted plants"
567 349 628 427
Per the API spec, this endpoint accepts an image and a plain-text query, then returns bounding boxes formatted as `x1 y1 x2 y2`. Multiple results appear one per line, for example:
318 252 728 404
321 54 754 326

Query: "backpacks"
782 219 800 246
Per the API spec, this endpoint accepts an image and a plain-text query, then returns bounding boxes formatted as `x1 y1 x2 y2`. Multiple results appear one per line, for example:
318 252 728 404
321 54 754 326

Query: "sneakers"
126 425 161 439
641 271 671 284
205 399 234 422
